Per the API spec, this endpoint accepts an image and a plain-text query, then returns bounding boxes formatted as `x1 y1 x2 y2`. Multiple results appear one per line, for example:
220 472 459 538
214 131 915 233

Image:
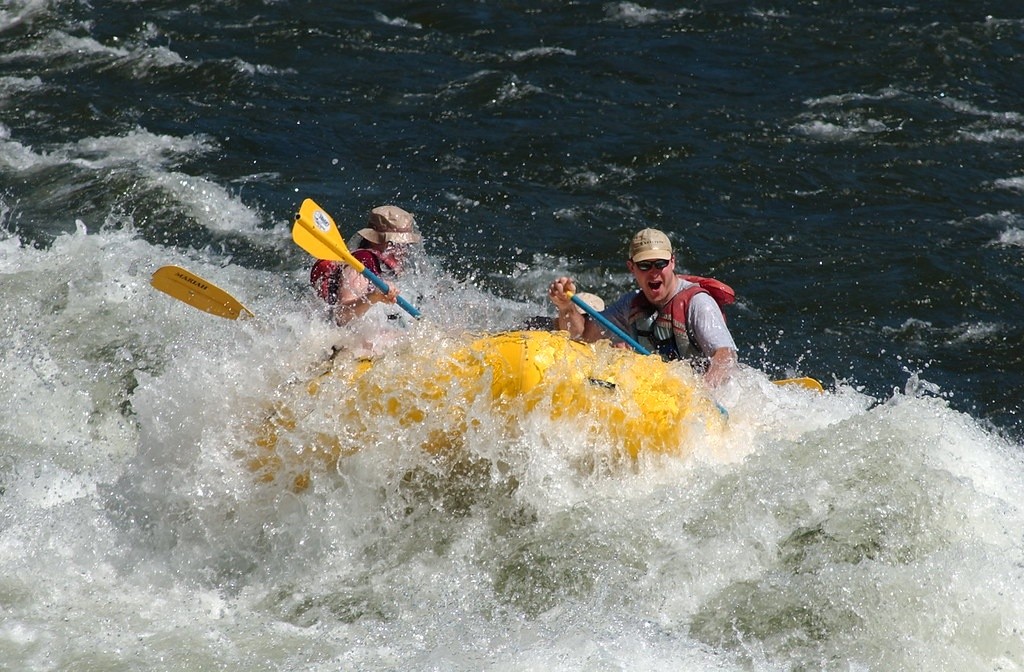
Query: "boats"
242 325 751 497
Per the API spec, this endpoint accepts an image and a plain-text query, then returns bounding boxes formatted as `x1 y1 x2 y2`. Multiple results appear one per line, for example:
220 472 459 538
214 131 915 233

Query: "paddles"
150 264 257 323
562 291 731 422
292 197 426 322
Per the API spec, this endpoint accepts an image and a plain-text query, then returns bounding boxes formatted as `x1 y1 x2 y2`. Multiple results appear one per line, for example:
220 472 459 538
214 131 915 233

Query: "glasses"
636 260 669 271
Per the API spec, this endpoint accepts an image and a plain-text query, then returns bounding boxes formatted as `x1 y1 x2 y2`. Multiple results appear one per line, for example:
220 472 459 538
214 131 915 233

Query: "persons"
333 206 413 328
548 228 738 385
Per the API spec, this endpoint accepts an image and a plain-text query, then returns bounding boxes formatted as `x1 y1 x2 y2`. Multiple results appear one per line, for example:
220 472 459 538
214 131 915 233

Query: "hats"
357 205 420 244
629 228 673 262
